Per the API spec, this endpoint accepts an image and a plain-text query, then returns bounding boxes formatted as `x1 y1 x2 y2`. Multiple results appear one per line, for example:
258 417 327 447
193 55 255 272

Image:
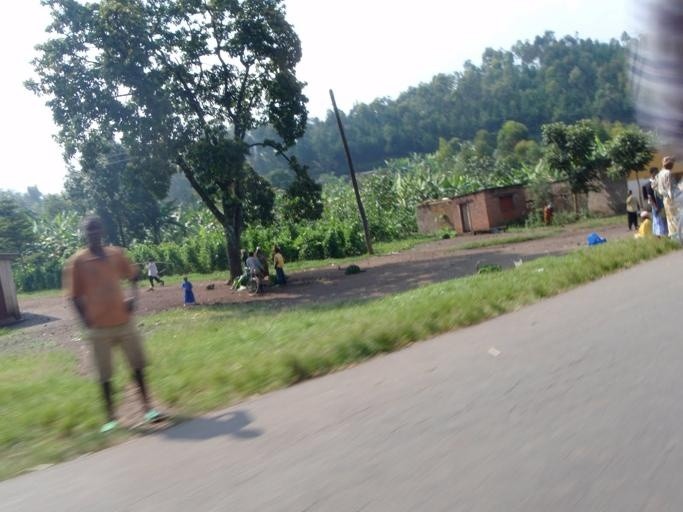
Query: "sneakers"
143 408 163 423
100 418 119 434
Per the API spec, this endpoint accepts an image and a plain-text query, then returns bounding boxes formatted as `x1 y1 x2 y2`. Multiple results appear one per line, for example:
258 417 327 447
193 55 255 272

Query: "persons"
181 276 196 307
227 244 287 294
543 203 553 227
623 153 682 243
59 214 169 437
143 256 164 291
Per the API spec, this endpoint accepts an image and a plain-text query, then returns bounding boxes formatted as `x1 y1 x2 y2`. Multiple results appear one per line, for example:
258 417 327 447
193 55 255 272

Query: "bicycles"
240 265 260 297
